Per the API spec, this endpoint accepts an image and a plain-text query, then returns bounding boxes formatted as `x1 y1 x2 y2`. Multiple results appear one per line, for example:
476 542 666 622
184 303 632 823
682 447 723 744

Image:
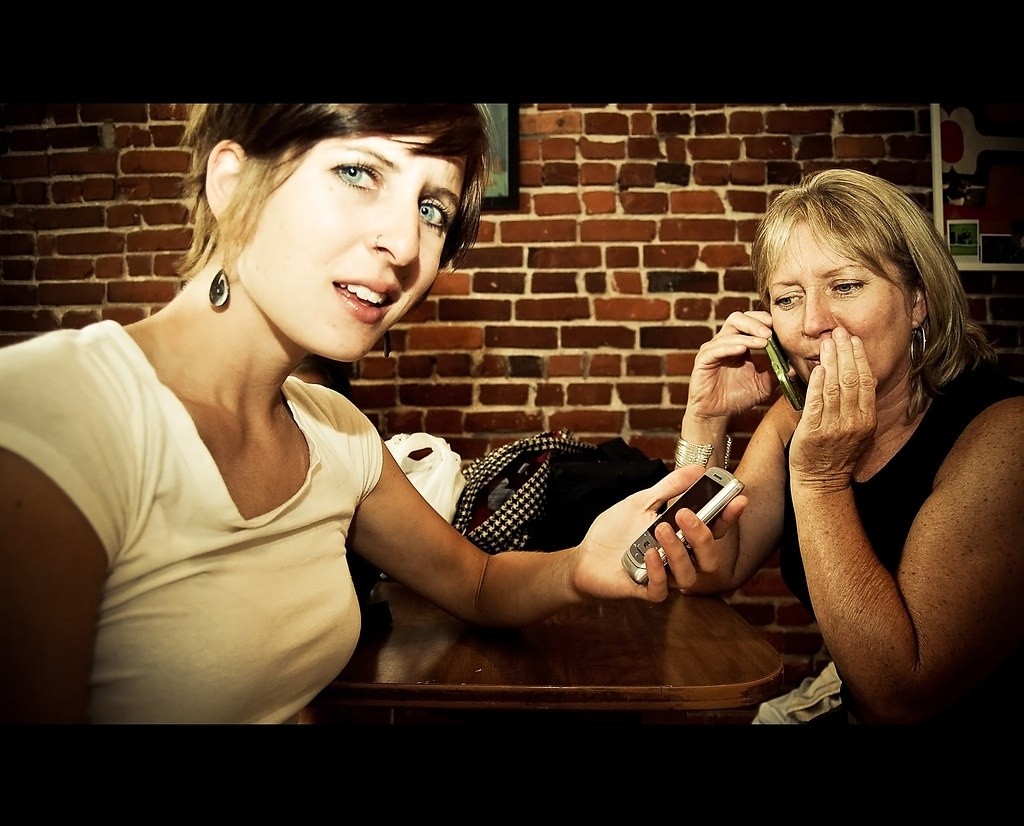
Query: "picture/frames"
472 103 520 211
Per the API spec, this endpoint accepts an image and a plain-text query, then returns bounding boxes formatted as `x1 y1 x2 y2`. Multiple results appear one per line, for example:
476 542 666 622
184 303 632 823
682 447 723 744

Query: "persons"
0 102 747 725
666 170 1024 725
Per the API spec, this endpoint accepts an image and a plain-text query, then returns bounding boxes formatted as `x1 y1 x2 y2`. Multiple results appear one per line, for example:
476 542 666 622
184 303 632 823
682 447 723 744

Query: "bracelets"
673 434 733 469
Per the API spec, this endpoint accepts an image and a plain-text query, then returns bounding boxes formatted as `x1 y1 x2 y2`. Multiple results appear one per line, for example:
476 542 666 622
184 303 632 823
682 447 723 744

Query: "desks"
332 572 784 826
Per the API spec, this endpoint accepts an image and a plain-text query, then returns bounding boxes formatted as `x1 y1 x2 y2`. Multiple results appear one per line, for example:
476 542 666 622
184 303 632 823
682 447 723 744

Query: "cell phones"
764 339 804 412
620 466 745 585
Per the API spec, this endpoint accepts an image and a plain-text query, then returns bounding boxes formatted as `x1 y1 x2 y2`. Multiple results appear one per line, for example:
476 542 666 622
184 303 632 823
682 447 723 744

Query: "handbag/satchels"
449 426 671 555
384 433 466 527
